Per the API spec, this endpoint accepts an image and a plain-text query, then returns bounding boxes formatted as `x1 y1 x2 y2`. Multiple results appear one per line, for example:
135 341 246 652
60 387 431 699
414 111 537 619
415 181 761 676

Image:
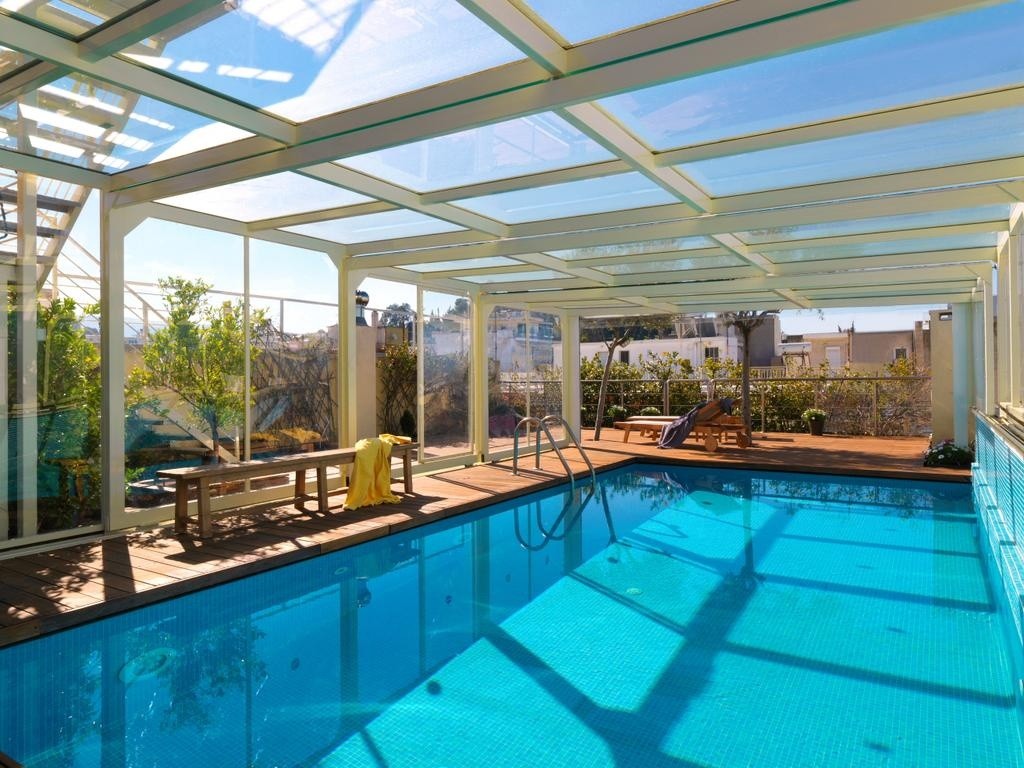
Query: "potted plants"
802 408 830 435
608 405 626 429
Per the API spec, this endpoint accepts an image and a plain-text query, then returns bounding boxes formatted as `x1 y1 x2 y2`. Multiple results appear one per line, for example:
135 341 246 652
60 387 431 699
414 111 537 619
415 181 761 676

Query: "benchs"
156 443 421 540
49 427 322 529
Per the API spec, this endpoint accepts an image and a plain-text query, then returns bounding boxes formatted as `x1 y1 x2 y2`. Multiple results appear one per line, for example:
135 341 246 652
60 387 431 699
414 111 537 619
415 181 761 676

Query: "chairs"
614 398 751 452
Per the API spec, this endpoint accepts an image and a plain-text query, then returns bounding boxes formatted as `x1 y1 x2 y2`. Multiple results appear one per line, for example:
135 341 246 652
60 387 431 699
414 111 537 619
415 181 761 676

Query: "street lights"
495 310 507 361
